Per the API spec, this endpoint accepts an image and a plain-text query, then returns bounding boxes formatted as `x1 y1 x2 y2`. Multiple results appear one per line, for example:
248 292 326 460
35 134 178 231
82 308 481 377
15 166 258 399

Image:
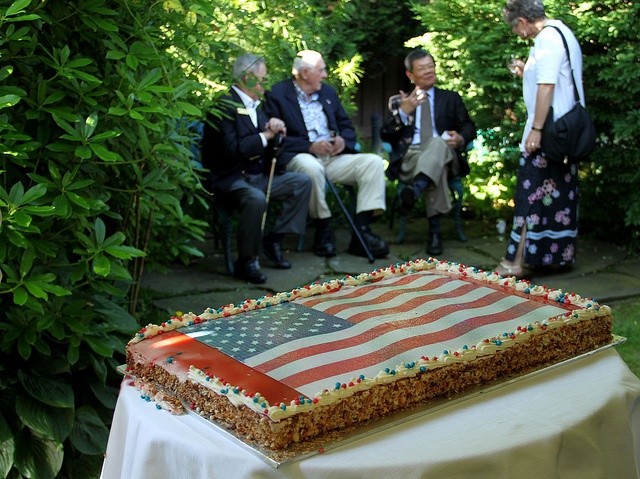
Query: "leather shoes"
399 187 415 217
313 229 337 256
426 233 443 255
234 260 266 283
263 237 291 267
349 226 390 256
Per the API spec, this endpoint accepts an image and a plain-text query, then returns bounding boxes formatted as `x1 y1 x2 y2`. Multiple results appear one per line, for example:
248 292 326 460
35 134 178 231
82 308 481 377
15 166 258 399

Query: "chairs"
175 122 239 276
383 141 473 244
295 142 361 251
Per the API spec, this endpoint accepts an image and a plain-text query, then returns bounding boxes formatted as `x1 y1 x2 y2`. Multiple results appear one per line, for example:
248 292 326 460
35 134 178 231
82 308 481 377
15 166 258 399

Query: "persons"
379 51 478 256
200 54 311 283
262 50 389 257
491 0 587 277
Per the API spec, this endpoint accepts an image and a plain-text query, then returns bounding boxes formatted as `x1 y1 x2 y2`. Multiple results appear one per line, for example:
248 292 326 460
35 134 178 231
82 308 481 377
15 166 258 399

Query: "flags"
175 269 572 402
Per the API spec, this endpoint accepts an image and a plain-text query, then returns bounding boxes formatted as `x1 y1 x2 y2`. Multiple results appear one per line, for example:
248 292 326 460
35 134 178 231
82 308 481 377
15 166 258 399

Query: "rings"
531 143 535 146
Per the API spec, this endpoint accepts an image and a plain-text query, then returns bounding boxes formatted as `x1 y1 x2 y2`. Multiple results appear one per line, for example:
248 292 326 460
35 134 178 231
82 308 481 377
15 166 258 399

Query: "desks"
100 347 640 479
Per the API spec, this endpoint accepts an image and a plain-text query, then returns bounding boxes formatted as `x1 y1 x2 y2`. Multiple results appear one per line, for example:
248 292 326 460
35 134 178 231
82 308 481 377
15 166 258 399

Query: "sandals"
494 257 523 276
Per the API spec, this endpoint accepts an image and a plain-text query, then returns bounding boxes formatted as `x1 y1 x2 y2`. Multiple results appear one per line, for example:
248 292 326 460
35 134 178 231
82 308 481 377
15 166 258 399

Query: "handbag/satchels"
540 103 596 168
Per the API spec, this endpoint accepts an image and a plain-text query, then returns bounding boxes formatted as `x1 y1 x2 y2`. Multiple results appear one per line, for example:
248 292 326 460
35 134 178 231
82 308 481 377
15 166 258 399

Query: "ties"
420 93 433 146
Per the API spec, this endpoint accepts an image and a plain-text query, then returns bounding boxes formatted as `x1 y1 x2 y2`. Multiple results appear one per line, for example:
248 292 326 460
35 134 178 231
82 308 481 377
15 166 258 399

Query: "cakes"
124 256 614 451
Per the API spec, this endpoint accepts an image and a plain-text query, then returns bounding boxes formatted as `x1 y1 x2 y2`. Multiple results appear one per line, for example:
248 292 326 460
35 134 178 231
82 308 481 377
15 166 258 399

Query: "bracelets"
532 126 544 131
